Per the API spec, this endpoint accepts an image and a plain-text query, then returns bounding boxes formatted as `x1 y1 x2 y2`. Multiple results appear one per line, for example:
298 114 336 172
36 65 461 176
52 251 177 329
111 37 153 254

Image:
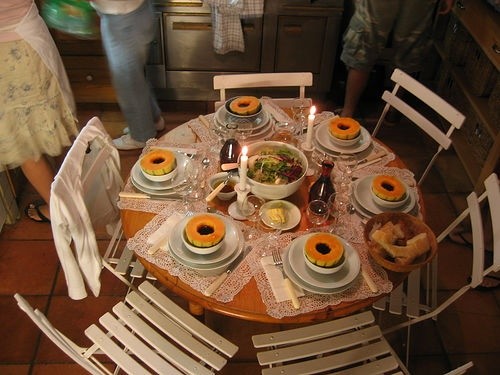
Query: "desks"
120 108 426 325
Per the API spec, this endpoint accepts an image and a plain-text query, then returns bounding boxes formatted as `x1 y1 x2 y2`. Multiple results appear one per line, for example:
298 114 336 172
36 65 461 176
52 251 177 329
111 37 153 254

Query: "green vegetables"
247 148 305 184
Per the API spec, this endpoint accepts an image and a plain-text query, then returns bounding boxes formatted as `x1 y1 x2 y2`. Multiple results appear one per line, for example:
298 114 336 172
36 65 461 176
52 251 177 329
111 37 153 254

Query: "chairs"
15 68 500 375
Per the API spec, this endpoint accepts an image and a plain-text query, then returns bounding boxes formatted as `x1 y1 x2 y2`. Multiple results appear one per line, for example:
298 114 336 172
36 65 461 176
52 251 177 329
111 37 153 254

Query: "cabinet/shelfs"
36 0 118 103
416 0 500 225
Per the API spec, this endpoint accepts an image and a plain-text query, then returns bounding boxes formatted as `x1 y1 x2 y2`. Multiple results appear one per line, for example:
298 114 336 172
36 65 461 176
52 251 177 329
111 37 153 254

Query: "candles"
240 145 248 191
306 106 316 148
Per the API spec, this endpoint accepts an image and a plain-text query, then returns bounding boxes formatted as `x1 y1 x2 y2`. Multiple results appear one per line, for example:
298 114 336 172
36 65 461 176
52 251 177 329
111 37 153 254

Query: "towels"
205 0 264 55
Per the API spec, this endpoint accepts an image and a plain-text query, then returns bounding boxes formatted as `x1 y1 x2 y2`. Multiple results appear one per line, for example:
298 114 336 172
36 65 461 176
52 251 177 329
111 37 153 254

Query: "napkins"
261 255 305 303
352 147 381 172
146 211 185 252
262 104 285 125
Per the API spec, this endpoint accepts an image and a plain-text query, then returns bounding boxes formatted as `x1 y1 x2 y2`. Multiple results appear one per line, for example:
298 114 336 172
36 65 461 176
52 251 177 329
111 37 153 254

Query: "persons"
89 0 165 150
0 0 78 222
447 209 500 290
329 0 458 135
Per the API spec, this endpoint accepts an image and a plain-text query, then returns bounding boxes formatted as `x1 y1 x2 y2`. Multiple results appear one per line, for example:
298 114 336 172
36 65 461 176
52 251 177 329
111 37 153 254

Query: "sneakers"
123 117 164 133
112 133 147 149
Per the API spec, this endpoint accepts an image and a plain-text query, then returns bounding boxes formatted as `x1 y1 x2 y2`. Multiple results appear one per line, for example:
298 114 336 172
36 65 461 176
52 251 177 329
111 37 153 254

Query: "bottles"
308 159 335 218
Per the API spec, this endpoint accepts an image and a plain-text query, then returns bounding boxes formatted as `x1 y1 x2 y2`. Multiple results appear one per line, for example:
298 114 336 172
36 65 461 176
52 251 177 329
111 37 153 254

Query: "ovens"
164 13 263 102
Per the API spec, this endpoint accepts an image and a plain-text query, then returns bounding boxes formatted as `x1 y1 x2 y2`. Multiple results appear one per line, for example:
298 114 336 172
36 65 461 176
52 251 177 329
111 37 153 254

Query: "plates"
167 212 246 271
212 95 273 138
129 149 197 198
348 172 416 220
313 117 371 158
259 199 301 231
281 232 362 295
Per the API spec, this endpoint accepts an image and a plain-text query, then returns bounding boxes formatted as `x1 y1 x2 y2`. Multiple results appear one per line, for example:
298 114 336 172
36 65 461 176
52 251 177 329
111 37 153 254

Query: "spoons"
200 157 210 189
269 248 300 309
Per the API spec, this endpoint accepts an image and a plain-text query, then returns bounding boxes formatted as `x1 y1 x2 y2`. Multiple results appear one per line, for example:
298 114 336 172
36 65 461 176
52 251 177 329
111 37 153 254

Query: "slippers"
25 203 51 222
445 229 493 253
466 274 500 291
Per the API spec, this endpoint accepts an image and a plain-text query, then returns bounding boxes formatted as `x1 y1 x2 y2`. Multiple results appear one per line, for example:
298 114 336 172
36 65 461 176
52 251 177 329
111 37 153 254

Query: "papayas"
230 96 261 116
304 233 344 268
185 214 225 248
140 149 177 175
371 175 406 202
329 117 361 140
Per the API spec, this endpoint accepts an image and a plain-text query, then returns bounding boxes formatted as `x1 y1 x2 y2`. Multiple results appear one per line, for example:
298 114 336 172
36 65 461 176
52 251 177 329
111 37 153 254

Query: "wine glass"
171 150 357 257
291 99 312 143
275 120 297 145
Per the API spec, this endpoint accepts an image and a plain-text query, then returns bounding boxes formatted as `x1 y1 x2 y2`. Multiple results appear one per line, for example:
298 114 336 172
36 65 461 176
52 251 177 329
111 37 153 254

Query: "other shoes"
383 109 403 126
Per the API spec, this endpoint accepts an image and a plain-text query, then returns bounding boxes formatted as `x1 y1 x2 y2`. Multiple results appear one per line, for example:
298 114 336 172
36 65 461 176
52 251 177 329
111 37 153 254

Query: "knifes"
203 246 251 297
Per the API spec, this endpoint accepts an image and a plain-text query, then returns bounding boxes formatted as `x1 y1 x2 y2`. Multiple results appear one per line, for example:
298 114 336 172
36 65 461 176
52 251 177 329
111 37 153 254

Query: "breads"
370 220 431 266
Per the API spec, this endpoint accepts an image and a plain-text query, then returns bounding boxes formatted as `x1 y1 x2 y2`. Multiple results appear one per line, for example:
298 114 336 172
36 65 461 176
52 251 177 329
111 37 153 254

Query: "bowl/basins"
238 140 309 200
140 163 178 183
363 211 438 273
221 97 263 123
206 169 239 200
368 182 411 210
180 221 225 254
327 128 362 148
302 244 348 274
